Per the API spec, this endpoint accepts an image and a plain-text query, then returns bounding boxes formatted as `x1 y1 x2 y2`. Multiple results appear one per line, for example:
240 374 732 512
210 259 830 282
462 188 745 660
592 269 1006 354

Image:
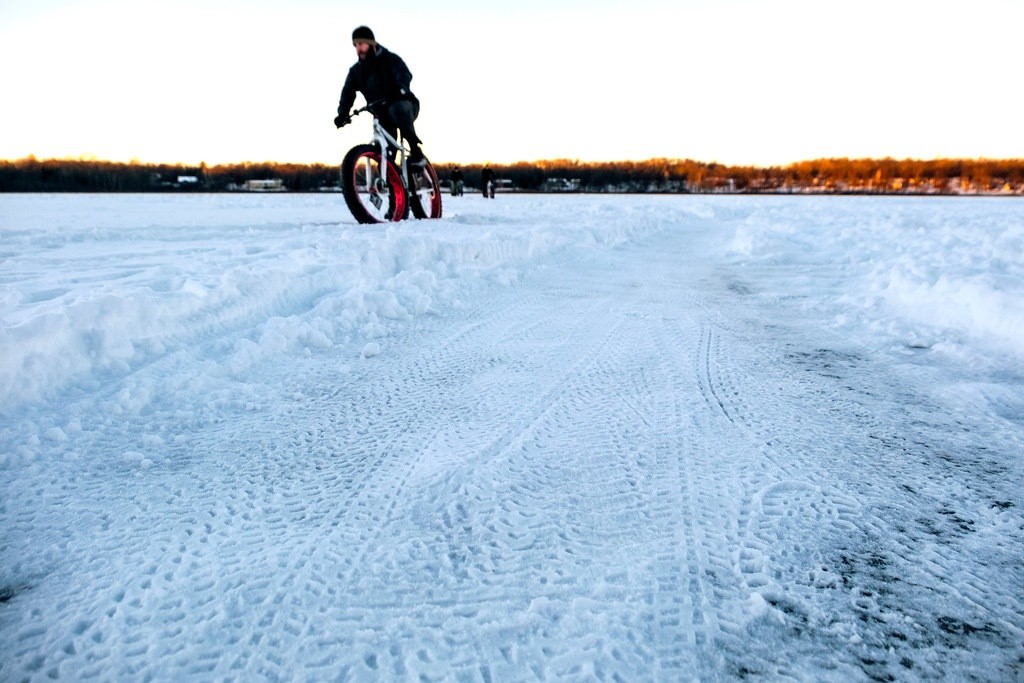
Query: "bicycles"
482 179 495 198
448 179 463 196
337 89 443 225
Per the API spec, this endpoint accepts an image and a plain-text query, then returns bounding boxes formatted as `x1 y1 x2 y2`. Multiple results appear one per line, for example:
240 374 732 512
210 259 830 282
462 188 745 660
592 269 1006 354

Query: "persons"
338 26 426 168
482 164 496 198
450 167 465 196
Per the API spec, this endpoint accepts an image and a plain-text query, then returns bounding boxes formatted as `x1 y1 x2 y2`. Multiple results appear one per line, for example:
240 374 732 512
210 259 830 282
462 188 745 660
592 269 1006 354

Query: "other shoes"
411 154 428 168
385 211 408 219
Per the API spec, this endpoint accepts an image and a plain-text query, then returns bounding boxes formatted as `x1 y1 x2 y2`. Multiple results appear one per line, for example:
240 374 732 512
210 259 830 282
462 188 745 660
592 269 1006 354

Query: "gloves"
335 113 352 129
396 82 407 95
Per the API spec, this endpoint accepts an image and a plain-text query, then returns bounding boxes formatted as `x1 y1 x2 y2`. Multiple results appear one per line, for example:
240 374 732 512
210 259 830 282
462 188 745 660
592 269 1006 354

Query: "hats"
352 26 376 46
